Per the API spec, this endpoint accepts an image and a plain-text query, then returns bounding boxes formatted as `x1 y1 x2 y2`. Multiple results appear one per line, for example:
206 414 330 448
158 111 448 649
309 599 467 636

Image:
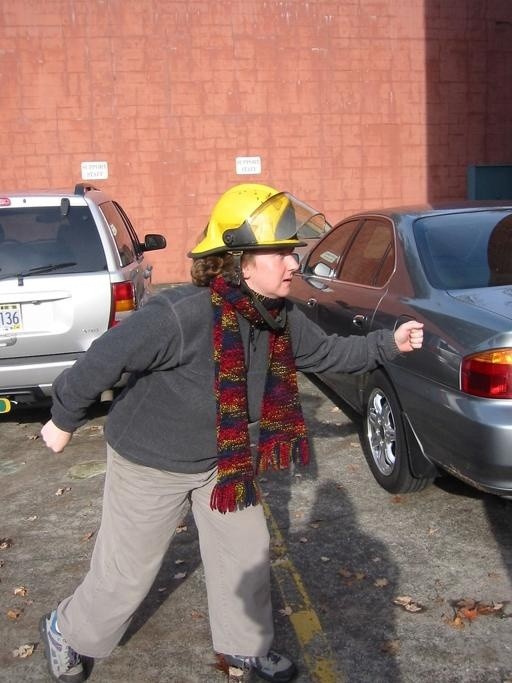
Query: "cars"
287 201 511 505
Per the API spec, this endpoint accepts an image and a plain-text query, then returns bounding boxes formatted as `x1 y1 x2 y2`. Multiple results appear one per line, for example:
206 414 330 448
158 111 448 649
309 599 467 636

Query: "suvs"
1 181 166 417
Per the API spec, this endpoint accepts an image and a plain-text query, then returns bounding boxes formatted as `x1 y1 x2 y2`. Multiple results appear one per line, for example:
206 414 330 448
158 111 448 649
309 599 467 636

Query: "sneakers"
222 648 295 683
37 607 86 683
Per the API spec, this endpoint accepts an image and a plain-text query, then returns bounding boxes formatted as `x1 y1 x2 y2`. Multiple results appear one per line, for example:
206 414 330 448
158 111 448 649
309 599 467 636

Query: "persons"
36 181 425 683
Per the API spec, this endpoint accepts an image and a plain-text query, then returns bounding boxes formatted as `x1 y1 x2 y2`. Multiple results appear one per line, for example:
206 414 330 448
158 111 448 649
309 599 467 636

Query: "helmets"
186 181 328 260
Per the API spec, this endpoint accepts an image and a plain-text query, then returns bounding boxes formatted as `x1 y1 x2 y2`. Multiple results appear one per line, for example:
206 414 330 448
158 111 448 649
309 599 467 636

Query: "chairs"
0 222 99 275
